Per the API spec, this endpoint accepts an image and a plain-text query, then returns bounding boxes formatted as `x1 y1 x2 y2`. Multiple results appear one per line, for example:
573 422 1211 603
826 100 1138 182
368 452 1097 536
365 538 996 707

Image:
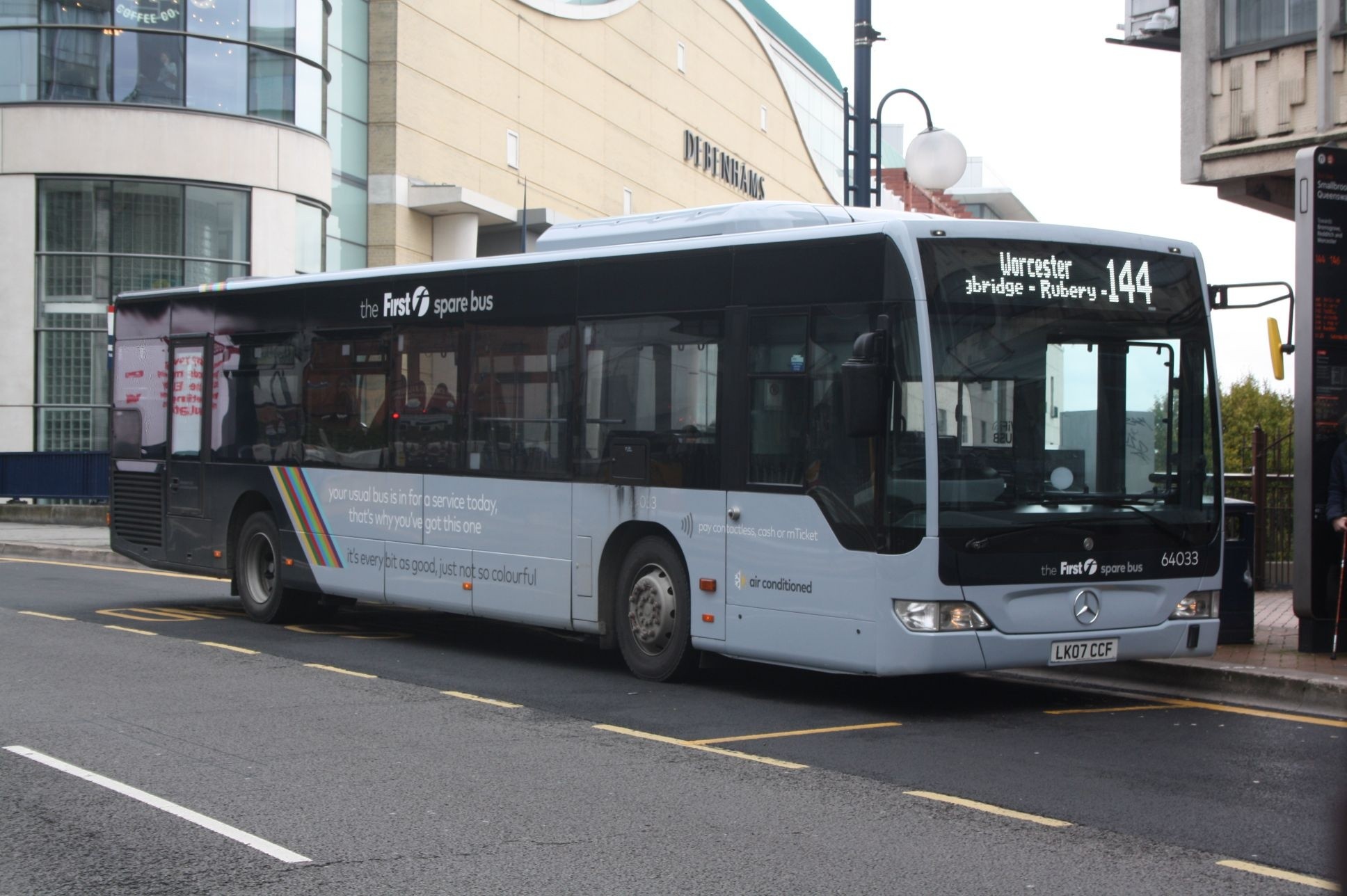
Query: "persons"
157 50 178 90
1325 412 1347 533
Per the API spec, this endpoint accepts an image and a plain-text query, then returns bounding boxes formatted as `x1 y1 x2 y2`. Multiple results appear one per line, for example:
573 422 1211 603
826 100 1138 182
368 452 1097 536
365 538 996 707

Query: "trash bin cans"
1216 496 1256 647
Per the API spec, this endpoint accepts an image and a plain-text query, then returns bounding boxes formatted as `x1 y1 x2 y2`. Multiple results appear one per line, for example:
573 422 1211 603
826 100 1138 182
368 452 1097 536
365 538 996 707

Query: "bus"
107 201 1297 685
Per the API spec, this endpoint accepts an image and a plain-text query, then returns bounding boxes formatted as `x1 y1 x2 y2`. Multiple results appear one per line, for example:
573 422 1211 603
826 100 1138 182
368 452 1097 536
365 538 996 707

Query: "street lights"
843 86 967 208
